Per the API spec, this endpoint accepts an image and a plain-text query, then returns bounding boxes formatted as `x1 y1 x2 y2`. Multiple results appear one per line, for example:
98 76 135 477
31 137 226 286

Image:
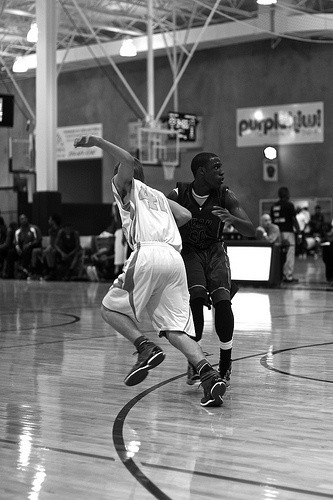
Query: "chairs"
25 236 116 285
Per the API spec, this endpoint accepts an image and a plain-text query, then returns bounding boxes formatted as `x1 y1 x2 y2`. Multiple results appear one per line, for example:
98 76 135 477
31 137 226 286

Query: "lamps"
120 32 137 58
12 53 28 74
25 18 39 43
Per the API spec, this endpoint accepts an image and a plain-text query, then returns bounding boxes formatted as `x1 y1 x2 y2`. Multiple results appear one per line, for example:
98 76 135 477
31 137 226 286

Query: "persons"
256 209 285 292
75 130 226 410
162 152 257 390
266 181 332 284
0 212 127 281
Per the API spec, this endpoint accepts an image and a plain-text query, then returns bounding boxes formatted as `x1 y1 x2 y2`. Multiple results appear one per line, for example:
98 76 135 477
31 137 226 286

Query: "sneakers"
123 342 165 387
199 369 226 407
86 266 104 281
186 362 200 385
218 359 232 388
283 277 299 283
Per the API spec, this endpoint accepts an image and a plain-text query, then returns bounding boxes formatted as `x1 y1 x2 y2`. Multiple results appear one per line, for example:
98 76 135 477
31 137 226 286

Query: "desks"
225 238 290 290
